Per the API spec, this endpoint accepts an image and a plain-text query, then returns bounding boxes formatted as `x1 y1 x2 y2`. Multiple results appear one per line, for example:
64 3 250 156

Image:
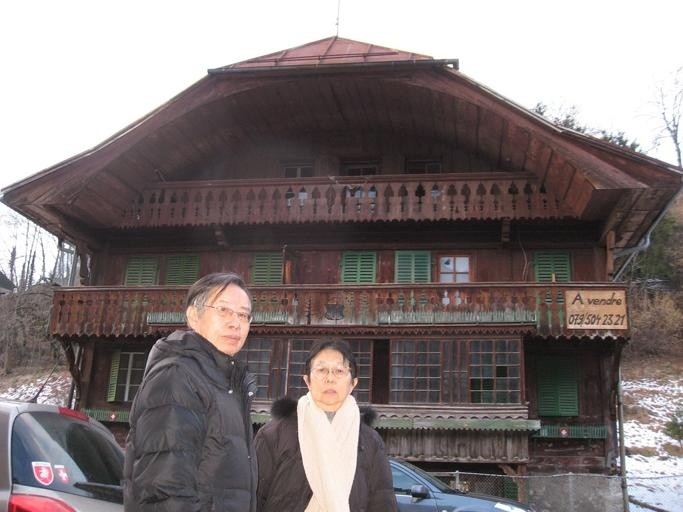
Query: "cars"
0 402 124 512
389 457 534 511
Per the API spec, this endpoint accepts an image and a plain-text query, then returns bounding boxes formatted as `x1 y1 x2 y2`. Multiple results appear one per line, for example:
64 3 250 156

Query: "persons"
120 272 257 511
252 336 397 512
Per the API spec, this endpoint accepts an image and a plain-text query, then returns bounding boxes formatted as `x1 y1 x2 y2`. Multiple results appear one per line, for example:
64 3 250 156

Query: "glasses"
309 367 351 379
198 303 254 325
